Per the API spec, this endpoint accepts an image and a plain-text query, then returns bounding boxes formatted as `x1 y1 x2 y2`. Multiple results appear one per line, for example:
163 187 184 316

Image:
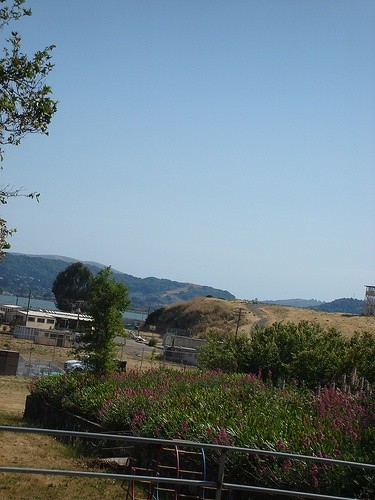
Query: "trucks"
64 355 128 375
